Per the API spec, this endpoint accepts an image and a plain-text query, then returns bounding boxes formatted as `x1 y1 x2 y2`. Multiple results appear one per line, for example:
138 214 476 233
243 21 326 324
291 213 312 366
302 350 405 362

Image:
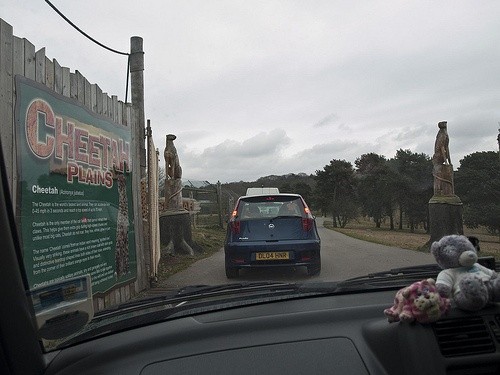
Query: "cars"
224 193 322 277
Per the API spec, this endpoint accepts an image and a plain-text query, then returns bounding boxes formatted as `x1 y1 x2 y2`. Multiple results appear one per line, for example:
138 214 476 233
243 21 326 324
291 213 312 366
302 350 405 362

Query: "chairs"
242 205 260 218
278 203 300 216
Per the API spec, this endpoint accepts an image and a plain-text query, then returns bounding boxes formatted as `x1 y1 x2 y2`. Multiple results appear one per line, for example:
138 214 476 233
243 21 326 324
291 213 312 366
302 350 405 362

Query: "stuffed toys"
383 232 500 326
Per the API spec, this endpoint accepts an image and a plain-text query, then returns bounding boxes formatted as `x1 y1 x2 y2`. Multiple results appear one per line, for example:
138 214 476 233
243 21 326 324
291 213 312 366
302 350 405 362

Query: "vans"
245 188 283 215
